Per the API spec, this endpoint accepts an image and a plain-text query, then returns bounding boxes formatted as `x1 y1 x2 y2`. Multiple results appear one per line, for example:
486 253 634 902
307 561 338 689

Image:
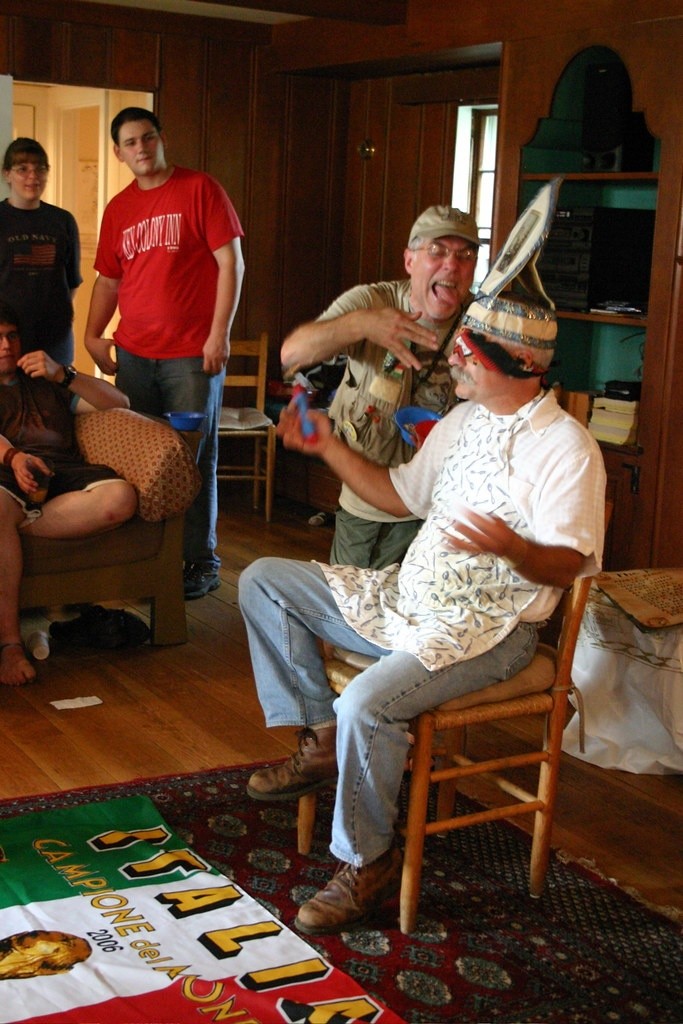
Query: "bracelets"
3 448 20 466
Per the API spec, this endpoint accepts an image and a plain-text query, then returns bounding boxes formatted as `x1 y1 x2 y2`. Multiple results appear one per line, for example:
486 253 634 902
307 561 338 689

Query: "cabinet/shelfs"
512 165 665 461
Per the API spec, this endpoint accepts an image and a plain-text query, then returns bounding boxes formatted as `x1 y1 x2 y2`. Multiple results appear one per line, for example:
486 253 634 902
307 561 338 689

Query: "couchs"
22 407 204 649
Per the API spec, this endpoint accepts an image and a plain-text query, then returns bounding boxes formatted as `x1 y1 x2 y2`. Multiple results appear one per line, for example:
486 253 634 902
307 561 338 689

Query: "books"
562 380 642 446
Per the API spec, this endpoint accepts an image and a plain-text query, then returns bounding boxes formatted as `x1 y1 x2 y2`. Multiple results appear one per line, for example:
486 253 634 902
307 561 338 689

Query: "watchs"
60 365 77 388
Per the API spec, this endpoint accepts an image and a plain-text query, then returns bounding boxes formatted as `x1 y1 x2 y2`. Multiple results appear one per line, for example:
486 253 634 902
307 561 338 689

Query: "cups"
25 630 50 659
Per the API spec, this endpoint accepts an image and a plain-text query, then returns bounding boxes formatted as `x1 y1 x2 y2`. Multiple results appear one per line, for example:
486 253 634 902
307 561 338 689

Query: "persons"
84 108 245 598
280 206 483 571
238 297 607 936
0 299 138 685
0 137 83 366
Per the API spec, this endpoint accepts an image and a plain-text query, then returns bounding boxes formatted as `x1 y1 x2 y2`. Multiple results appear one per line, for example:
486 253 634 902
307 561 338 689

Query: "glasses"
410 242 478 267
8 165 48 177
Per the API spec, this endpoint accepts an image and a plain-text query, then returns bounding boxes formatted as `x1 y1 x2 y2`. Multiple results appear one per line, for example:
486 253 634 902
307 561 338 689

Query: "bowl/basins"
163 411 209 431
394 406 442 447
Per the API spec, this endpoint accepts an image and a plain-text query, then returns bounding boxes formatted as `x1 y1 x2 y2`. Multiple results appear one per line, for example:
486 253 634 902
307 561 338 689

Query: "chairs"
295 495 615 937
214 330 279 524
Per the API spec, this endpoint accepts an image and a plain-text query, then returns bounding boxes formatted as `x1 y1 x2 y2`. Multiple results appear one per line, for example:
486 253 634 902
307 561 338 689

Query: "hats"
409 205 481 249
460 176 564 349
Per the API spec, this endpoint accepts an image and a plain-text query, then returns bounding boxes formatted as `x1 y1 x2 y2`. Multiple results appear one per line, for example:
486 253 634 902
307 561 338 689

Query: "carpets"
0 756 683 1024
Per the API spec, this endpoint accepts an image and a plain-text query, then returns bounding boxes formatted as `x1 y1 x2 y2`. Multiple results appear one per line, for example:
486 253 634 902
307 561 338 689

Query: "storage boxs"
562 390 603 428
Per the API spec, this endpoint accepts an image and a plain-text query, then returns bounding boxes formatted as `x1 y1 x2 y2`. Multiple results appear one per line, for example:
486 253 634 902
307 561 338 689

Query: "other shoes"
48 604 151 653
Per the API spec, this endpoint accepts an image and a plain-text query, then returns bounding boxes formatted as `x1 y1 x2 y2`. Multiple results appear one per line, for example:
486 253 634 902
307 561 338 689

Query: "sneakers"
247 725 339 802
295 845 404 937
182 562 221 600
